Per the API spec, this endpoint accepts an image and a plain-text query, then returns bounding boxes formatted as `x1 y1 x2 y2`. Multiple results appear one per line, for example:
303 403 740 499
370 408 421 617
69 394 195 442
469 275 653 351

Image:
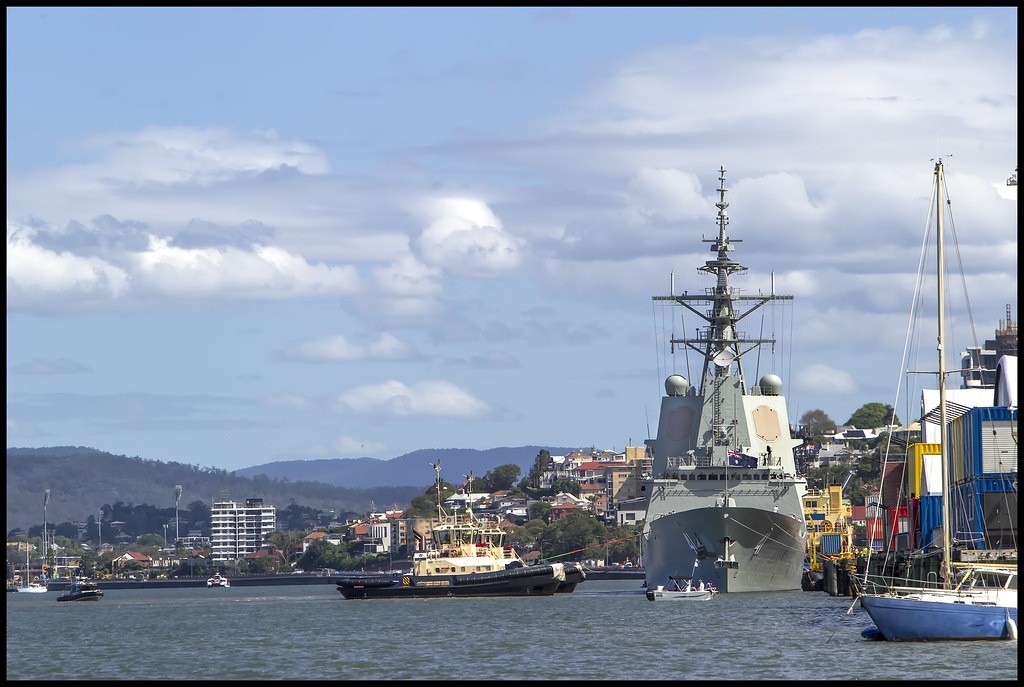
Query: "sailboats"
16 543 47 594
844 152 1018 644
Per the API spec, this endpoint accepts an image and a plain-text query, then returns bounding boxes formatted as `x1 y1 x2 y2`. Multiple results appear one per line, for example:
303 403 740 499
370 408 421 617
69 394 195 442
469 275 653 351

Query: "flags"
727 449 742 459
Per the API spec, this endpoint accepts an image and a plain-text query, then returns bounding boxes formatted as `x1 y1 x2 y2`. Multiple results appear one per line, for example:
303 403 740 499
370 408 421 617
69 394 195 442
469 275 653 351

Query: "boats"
56 561 104 602
646 557 720 602
335 457 587 600
640 162 808 603
206 567 230 588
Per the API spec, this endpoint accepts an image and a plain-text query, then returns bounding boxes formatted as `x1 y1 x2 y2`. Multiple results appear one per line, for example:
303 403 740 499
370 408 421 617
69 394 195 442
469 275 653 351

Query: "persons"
674 579 713 594
773 505 779 513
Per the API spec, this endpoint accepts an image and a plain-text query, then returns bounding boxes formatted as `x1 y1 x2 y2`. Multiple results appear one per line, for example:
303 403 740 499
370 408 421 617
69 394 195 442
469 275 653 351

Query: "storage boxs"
820 405 1017 556
